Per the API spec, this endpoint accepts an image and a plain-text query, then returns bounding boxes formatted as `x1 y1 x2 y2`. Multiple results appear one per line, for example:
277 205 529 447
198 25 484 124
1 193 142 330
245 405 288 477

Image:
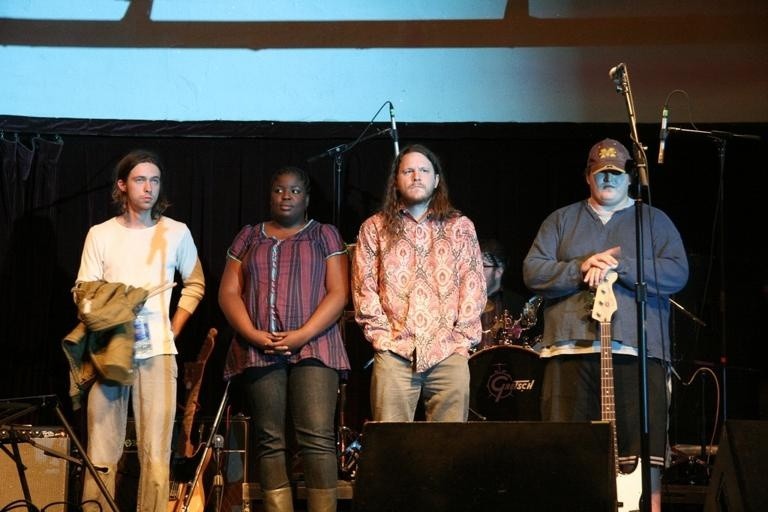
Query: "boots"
307 487 336 512
261 487 293 512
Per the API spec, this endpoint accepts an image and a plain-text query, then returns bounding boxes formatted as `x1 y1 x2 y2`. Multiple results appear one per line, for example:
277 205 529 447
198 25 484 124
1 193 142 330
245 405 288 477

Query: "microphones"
658 105 668 163
389 103 400 158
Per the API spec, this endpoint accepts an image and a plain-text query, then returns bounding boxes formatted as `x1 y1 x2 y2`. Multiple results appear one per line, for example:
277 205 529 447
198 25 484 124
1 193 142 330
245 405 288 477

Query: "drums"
670 356 722 456
468 343 544 421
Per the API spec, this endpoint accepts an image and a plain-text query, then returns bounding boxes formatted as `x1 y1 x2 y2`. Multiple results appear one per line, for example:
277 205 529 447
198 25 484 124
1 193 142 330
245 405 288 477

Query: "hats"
587 137 632 176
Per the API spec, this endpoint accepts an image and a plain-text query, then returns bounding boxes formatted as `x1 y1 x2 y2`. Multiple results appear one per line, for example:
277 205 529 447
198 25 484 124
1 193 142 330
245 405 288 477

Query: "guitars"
589 267 643 512
168 328 225 512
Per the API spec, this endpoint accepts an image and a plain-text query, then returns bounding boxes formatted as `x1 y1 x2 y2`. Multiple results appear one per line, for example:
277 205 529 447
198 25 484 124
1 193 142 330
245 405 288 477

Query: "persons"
480 233 538 348
217 166 353 512
69 150 206 512
350 144 488 422
521 137 690 511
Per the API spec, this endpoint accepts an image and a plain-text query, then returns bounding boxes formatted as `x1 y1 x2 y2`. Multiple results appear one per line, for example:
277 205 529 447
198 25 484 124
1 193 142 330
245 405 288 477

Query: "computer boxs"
668 360 761 471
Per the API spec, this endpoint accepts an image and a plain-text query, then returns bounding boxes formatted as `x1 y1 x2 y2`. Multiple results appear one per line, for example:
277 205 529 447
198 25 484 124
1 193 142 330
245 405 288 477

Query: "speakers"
0 425 71 512
349 421 618 512
70 417 249 512
